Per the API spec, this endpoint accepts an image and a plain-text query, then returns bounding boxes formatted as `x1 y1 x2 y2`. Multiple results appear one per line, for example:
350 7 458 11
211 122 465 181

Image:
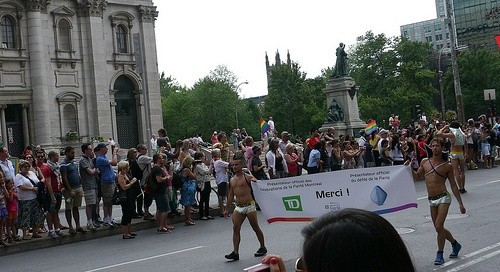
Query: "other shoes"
185 220 196 226
123 232 137 239
449 242 463 258
47 231 58 238
254 247 268 257
132 211 155 221
68 229 76 234
91 220 100 227
220 212 231 217
0 227 47 246
86 223 97 231
224 251 240 260
434 251 445 265
457 188 467 194
96 216 120 229
167 204 213 216
55 230 66 237
76 227 87 233
198 215 215 220
157 226 174 233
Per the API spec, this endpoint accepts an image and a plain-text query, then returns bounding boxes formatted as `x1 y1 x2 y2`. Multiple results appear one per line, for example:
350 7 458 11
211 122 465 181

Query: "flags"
365 119 378 135
258 117 271 138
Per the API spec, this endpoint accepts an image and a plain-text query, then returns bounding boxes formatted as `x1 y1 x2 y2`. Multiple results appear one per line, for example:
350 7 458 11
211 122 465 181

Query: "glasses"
26 157 32 159
37 156 44 158
87 148 93 152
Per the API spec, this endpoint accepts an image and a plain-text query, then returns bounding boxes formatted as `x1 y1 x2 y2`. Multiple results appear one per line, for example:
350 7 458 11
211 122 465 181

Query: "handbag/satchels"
111 186 126 206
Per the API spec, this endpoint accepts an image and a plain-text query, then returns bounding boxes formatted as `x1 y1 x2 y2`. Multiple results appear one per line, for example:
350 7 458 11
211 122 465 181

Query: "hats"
96 143 109 150
252 146 261 154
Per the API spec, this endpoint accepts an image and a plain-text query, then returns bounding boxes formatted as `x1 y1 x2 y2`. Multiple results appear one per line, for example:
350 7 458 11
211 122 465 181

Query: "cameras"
244 262 271 272
192 160 202 164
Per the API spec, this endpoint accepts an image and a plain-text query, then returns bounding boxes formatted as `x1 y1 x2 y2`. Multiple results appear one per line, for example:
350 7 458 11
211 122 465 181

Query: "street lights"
236 82 249 129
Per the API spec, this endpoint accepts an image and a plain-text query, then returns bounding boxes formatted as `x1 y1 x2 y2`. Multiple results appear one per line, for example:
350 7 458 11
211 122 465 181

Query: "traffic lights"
415 104 421 119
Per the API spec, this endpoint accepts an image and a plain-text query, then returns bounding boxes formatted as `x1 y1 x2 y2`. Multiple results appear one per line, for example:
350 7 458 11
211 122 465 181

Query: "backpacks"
172 167 191 189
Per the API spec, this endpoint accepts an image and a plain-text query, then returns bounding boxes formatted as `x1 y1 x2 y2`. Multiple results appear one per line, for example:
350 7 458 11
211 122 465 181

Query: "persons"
331 43 350 78
0 113 500 272
326 99 344 123
